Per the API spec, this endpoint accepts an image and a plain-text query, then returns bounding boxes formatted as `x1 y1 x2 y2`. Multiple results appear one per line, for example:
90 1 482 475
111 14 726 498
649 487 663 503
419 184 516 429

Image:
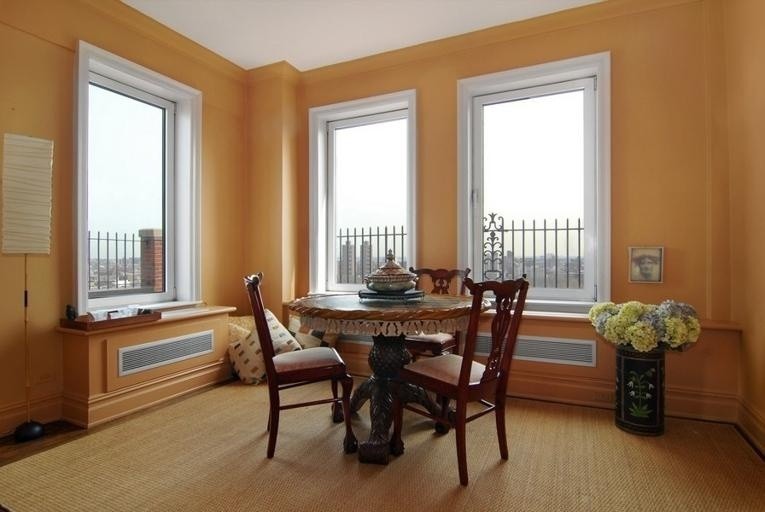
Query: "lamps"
0 131 55 442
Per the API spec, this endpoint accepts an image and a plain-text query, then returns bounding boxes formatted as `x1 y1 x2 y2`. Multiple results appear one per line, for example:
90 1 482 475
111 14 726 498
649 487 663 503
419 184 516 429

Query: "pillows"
226 308 301 386
289 326 340 349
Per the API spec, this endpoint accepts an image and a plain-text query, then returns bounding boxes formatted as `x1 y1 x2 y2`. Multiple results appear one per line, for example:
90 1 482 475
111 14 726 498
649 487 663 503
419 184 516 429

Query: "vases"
614 346 665 436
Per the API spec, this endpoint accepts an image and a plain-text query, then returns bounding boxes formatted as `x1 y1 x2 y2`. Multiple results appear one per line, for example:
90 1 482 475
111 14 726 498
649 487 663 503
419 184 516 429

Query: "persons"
632 255 660 280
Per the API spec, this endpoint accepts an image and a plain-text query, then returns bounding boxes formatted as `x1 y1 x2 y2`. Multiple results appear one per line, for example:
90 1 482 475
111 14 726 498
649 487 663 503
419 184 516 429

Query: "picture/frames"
627 245 663 285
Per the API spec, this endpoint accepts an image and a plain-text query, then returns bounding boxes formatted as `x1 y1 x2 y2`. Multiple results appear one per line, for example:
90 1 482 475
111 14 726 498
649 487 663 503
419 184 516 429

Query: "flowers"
588 296 701 354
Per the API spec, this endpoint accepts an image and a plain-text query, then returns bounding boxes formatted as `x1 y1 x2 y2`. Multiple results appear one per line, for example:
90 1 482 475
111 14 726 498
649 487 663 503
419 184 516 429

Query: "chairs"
244 272 357 458
389 266 530 487
